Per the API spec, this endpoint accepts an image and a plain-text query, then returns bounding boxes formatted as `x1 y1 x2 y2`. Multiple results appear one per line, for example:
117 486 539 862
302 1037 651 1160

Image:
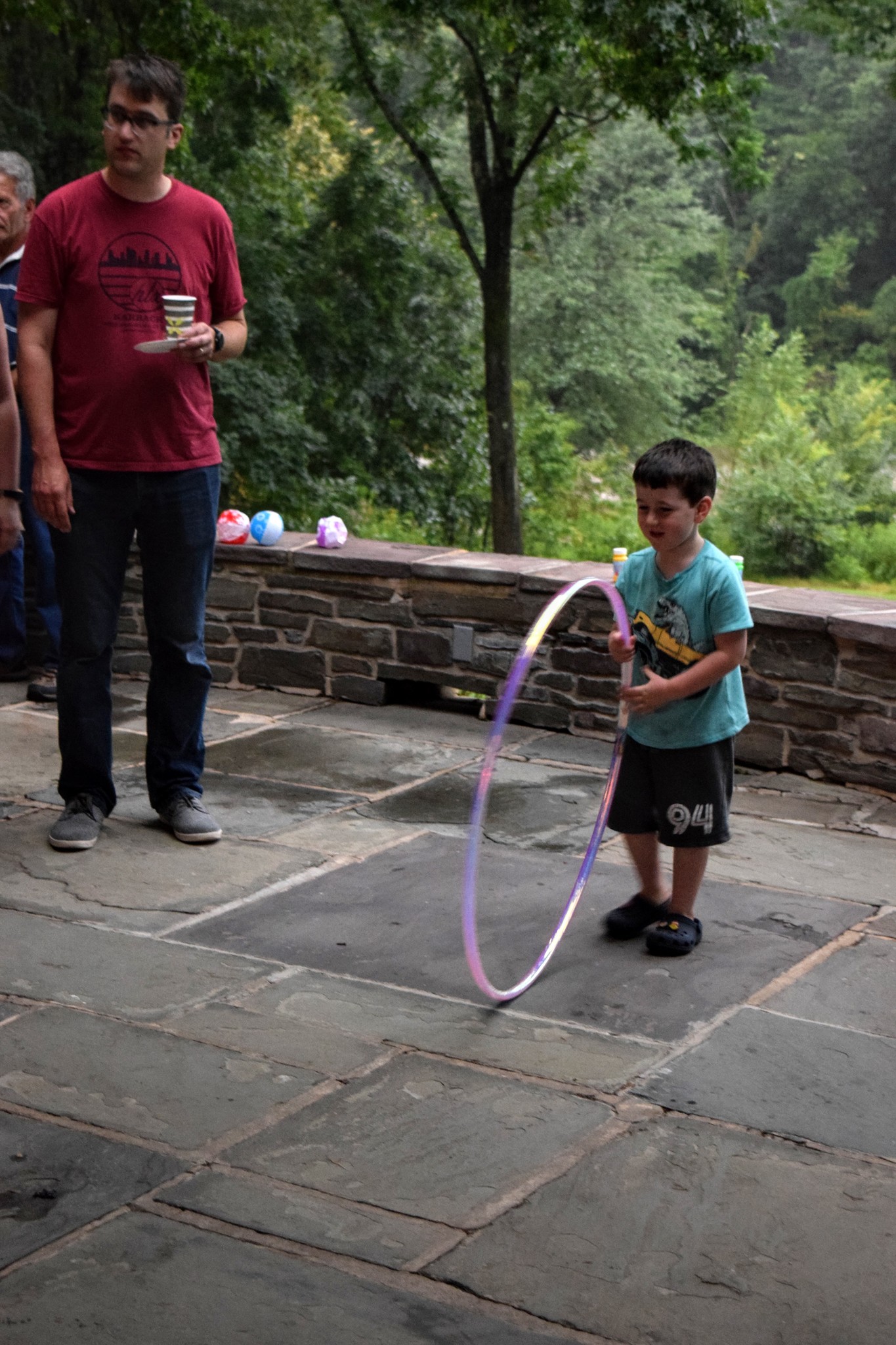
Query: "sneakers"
27 668 58 701
158 793 222 843
47 793 104 850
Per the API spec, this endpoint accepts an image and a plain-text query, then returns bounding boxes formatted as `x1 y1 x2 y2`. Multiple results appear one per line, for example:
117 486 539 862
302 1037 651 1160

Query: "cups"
161 294 198 339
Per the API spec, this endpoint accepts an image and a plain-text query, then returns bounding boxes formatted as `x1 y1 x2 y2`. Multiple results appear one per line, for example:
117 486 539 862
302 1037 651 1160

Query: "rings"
199 348 203 352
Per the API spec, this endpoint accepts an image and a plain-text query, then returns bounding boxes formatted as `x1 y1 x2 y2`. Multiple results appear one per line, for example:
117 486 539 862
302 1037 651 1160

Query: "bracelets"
0 488 25 501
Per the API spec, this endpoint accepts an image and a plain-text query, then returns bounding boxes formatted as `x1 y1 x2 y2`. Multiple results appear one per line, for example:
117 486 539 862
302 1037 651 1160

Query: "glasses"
100 102 177 139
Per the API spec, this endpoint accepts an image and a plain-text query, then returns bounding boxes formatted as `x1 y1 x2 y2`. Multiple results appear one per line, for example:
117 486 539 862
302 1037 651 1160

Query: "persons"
598 439 755 951
0 304 25 556
1 148 61 704
15 53 249 851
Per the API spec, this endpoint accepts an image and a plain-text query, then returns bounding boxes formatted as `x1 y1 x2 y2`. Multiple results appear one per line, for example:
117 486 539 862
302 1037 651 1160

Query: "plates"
134 338 188 353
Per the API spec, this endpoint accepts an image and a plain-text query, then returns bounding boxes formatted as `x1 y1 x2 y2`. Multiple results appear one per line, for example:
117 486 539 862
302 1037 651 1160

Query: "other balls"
250 510 284 546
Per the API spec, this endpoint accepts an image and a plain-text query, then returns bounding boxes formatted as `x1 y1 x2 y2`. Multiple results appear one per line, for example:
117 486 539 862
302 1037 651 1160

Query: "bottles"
613 547 628 584
730 555 744 579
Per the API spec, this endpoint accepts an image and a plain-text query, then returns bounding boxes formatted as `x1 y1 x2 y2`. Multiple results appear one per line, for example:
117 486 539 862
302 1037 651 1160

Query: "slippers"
645 912 702 957
603 892 672 941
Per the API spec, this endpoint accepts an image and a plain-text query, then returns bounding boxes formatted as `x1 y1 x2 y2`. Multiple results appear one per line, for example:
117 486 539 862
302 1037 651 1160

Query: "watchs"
212 328 224 353
